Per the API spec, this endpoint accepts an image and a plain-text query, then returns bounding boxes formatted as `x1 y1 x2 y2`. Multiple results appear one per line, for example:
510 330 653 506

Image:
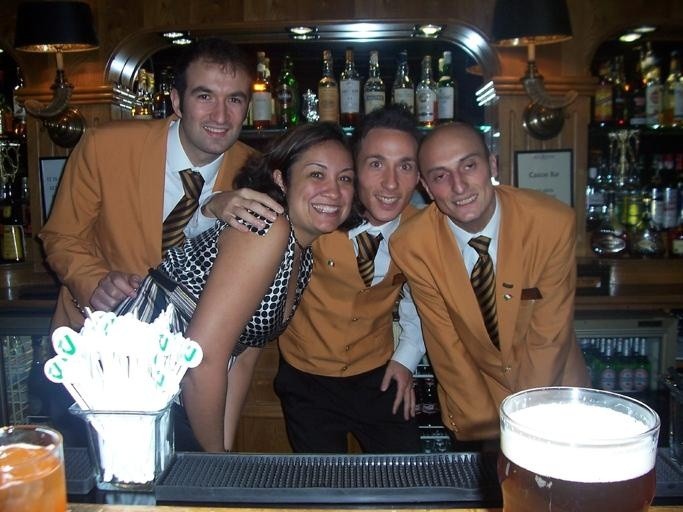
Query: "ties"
354 231 384 287
161 167 204 257
468 236 500 352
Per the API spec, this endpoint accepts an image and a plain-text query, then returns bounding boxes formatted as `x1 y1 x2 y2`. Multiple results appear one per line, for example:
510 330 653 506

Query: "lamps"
486 1 586 138
7 1 101 148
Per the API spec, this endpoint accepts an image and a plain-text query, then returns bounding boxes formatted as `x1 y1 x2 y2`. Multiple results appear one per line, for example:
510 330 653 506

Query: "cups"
69 397 179 491
488 386 660 512
0 423 67 512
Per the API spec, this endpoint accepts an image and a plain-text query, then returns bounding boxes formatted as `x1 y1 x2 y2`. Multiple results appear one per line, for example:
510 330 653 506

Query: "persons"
389 123 593 477
49 120 355 454
36 38 258 346
183 102 428 454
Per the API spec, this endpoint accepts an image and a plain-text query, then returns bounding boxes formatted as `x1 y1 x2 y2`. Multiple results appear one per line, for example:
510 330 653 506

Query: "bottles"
139 47 458 127
408 356 455 453
586 39 680 260
19 336 82 443
574 335 657 410
0 60 30 264
